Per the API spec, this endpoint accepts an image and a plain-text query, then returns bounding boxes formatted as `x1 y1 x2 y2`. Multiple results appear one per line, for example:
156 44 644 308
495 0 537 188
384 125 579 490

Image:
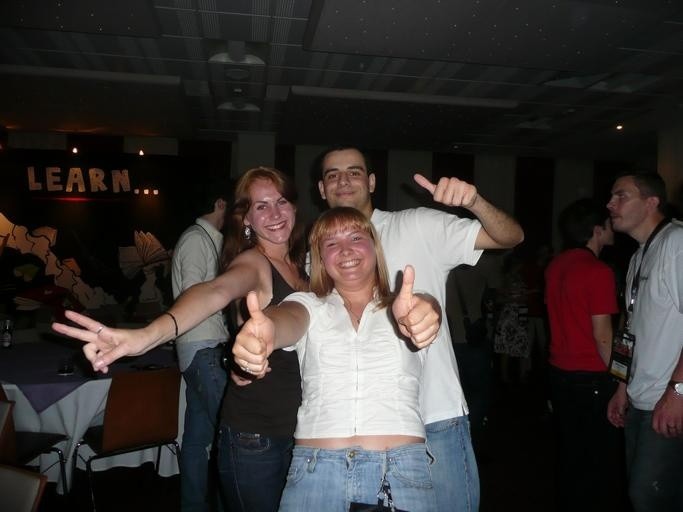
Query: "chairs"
72 365 181 512
0 467 47 511
0 400 16 440
0 385 69 497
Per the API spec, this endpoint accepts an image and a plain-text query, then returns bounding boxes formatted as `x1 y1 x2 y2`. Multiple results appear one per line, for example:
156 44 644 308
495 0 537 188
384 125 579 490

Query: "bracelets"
163 311 178 339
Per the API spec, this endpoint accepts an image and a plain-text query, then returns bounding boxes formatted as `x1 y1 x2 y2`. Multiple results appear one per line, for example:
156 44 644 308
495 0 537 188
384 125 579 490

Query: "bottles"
2 319 12 348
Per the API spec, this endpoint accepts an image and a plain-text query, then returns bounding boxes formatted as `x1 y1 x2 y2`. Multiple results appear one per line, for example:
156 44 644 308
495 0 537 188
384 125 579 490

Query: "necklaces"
349 309 362 324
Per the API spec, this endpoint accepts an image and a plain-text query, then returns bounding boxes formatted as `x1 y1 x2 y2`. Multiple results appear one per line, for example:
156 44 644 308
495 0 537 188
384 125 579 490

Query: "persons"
489 168 683 512
170 179 236 512
231 206 442 512
52 165 308 512
314 142 525 512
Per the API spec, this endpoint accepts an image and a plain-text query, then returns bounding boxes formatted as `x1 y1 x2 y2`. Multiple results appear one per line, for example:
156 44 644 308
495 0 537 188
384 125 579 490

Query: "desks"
0 340 187 495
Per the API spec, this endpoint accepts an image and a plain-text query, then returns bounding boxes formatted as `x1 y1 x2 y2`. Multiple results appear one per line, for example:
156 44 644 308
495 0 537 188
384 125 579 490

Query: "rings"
98 325 105 333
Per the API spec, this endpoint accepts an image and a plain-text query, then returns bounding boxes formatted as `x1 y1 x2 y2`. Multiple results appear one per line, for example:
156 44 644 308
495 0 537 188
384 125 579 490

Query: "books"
117 230 170 270
